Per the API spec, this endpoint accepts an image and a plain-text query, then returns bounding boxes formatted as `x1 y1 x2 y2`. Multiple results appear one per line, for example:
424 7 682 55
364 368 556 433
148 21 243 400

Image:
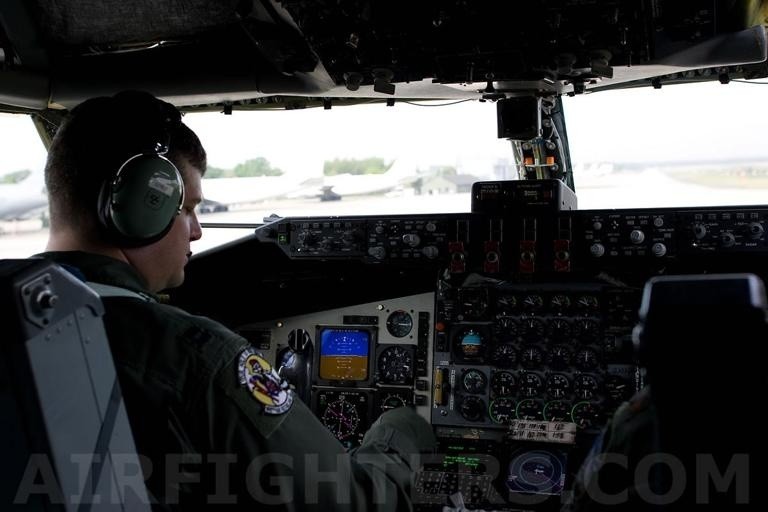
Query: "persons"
0 91 438 512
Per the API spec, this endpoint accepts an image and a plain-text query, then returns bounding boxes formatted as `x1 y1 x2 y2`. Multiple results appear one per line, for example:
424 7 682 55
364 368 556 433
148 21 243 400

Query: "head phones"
96 89 187 248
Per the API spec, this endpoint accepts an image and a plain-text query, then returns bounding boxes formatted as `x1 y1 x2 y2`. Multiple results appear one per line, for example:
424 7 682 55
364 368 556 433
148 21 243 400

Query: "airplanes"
2 155 423 230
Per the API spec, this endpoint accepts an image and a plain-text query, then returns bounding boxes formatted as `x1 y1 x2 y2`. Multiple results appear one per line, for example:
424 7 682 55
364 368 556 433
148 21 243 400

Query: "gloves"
362 406 437 472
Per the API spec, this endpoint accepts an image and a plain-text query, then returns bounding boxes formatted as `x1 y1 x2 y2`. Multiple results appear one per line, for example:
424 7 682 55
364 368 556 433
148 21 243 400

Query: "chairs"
0 260 152 512
561 272 768 511
